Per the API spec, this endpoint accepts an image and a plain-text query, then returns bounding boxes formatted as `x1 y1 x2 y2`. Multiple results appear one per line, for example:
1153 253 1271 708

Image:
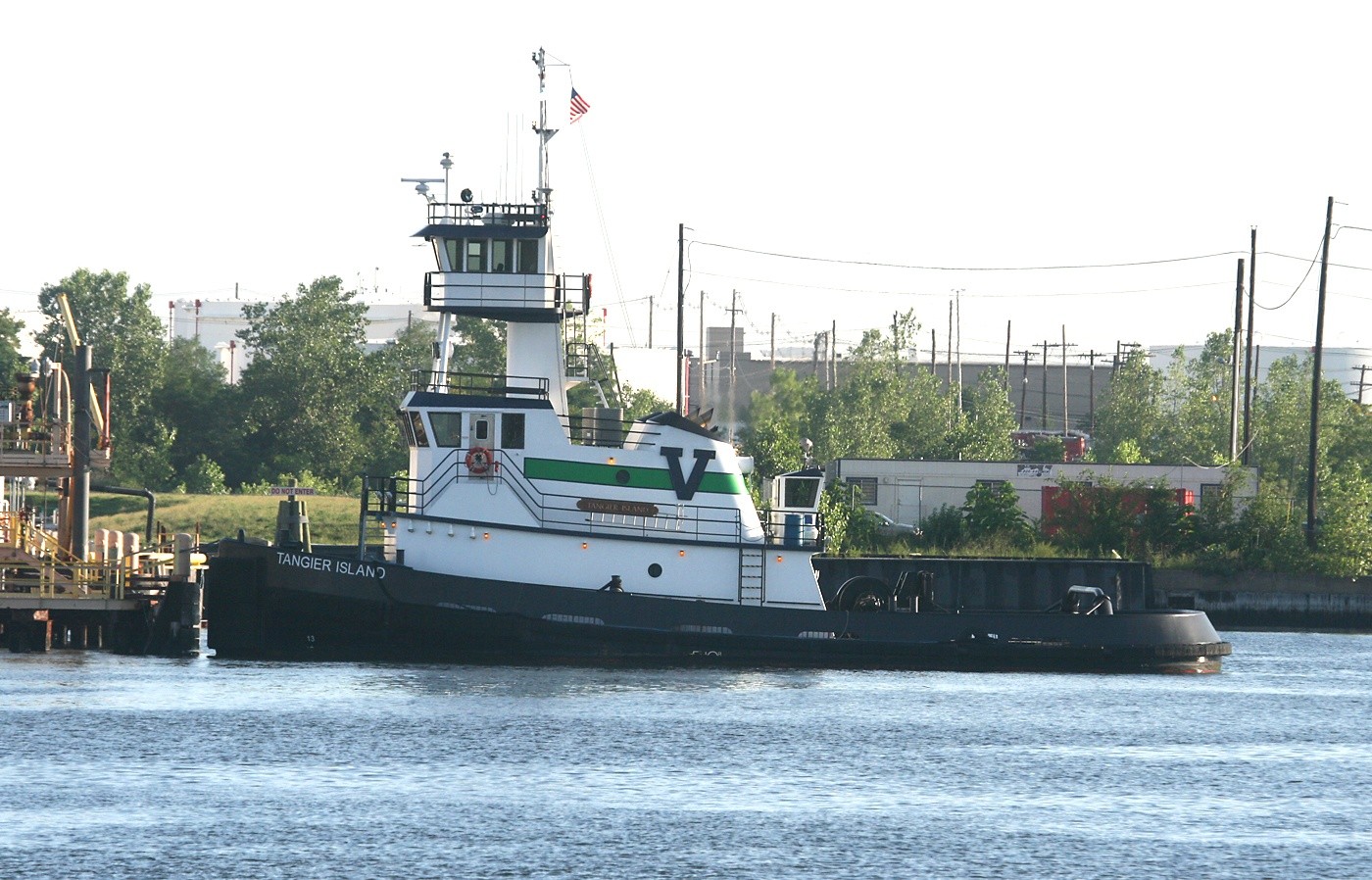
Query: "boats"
202 45 1233 683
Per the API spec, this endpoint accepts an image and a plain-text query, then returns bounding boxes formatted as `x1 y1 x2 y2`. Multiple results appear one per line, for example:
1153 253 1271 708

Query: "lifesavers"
464 446 492 475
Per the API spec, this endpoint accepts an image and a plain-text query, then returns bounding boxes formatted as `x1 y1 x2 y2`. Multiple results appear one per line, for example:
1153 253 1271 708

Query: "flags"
569 86 590 125
